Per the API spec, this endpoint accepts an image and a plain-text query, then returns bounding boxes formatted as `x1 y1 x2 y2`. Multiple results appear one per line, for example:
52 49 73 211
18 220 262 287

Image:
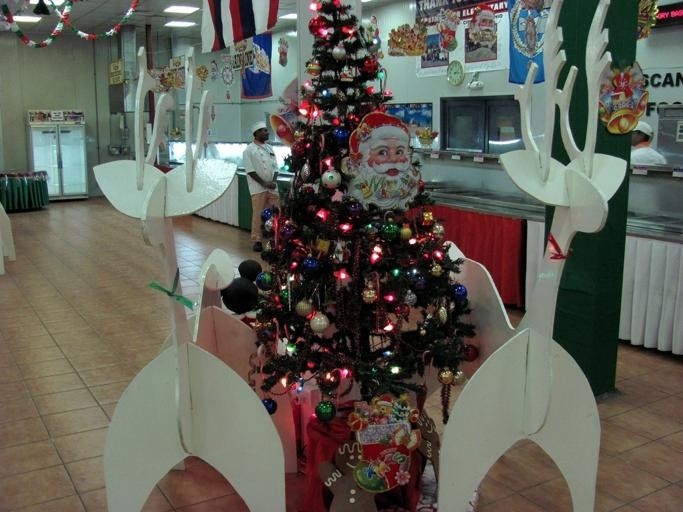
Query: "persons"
467 3 496 45
241 121 280 251
629 121 666 165
345 111 422 215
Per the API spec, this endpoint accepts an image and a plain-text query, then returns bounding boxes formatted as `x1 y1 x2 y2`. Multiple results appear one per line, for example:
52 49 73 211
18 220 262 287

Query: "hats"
632 121 652 137
252 121 267 137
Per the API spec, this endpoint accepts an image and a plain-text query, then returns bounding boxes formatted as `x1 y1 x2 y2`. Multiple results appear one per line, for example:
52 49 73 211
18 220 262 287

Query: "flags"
239 32 273 100
200 0 280 55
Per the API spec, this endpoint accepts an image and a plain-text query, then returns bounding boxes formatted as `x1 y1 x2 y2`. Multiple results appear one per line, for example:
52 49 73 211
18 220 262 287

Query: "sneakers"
253 241 262 252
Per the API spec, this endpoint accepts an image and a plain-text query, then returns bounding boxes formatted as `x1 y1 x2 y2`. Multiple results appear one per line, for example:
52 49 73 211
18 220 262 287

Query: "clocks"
445 61 465 87
